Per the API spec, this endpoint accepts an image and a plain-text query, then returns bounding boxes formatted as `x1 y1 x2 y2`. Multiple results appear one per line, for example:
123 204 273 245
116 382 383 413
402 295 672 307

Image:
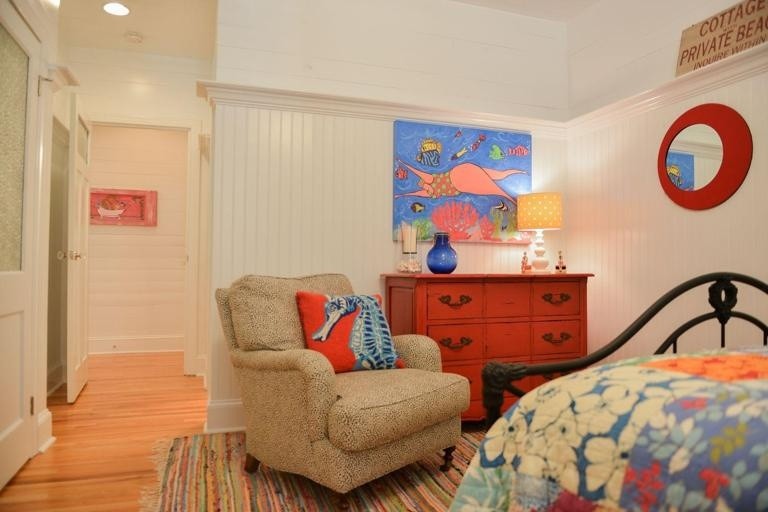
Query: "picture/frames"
89 188 158 227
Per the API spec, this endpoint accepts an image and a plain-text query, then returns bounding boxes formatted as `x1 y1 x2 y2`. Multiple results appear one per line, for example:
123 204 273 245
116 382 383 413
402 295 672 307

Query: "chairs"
216 272 472 498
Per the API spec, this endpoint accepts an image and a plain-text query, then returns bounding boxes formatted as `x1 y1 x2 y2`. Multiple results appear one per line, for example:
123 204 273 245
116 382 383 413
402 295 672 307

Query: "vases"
426 232 457 275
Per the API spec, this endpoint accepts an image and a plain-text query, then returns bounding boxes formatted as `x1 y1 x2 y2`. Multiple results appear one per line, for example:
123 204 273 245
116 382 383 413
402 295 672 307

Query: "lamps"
515 191 565 275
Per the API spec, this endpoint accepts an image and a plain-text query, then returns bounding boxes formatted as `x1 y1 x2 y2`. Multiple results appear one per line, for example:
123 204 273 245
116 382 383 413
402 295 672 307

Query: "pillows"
292 290 406 370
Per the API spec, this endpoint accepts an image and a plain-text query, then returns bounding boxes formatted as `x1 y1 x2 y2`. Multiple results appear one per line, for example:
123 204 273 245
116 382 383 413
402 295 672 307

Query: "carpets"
143 429 488 512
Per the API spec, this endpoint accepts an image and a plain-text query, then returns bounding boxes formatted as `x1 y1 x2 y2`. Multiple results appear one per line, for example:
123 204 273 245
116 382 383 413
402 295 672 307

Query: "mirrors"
658 103 753 210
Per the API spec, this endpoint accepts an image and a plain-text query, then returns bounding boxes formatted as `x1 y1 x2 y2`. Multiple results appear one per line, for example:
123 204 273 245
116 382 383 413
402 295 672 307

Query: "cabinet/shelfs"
380 270 596 424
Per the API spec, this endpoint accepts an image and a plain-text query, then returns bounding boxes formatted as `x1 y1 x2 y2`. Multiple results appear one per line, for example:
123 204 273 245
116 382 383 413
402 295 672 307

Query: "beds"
453 272 766 512
